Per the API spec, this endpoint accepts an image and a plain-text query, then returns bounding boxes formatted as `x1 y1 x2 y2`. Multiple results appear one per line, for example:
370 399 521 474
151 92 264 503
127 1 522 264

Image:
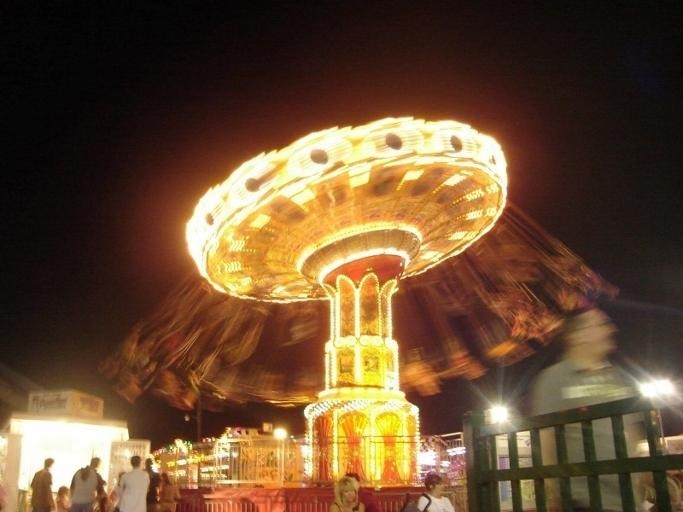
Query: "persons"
31 456 177 512
528 308 648 512
329 472 456 512
647 473 683 512
